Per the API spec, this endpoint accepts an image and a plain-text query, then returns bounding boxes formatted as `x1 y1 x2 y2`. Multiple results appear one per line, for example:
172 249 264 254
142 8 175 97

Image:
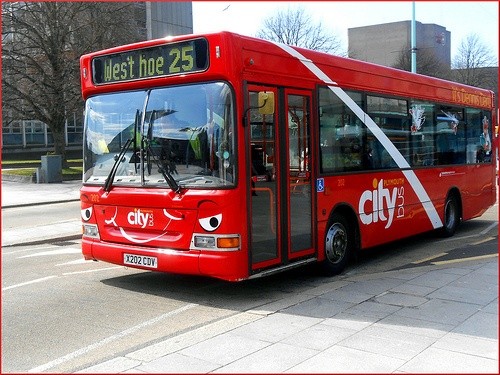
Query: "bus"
321 107 479 176
79 30 498 287
